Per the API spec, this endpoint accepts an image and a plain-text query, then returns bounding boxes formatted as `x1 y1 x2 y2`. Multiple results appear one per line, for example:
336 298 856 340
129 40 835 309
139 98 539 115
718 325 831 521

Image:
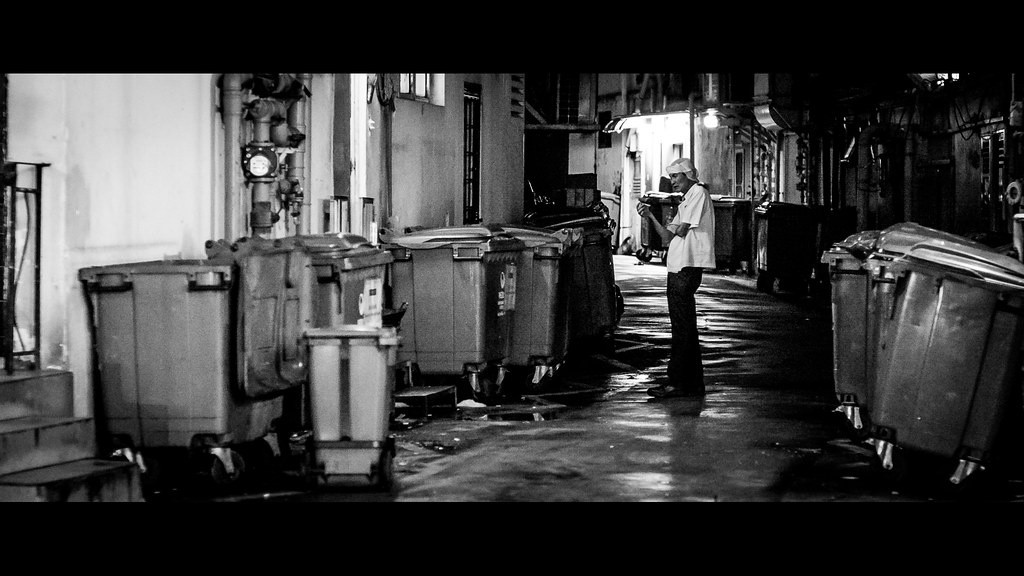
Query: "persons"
638 157 718 405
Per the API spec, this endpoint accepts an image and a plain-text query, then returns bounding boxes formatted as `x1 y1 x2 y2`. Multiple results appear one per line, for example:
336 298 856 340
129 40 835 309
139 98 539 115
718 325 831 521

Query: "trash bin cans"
486 223 585 402
378 224 495 402
659 192 685 267
708 194 752 274
543 216 618 357
819 230 881 441
77 235 310 504
302 323 404 492
636 191 671 264
753 200 816 303
272 231 395 479
860 221 1024 504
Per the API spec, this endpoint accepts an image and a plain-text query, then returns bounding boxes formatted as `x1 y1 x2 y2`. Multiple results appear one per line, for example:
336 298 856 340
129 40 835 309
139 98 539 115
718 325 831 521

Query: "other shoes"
668 397 701 415
646 383 682 398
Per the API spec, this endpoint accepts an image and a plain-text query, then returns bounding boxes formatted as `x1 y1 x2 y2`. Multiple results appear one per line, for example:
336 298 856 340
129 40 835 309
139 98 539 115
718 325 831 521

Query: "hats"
666 158 699 183
